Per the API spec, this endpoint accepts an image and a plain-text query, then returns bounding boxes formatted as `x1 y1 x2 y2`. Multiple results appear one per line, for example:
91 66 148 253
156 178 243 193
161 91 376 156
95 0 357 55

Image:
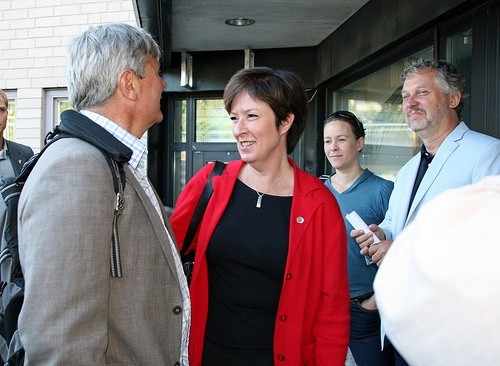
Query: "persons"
169 67 350 366
323 110 393 366
5 23 192 366
351 58 500 267
0 89 34 232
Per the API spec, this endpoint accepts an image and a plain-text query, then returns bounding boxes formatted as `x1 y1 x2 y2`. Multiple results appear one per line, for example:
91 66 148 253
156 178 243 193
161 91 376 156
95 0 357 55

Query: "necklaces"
246 164 290 208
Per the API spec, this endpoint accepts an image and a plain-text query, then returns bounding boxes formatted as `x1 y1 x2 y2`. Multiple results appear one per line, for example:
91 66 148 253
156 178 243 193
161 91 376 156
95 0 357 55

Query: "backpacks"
0 109 132 366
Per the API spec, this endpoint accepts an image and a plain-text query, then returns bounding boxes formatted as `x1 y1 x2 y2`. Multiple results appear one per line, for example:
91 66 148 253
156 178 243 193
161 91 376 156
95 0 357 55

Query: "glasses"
326 110 363 136
